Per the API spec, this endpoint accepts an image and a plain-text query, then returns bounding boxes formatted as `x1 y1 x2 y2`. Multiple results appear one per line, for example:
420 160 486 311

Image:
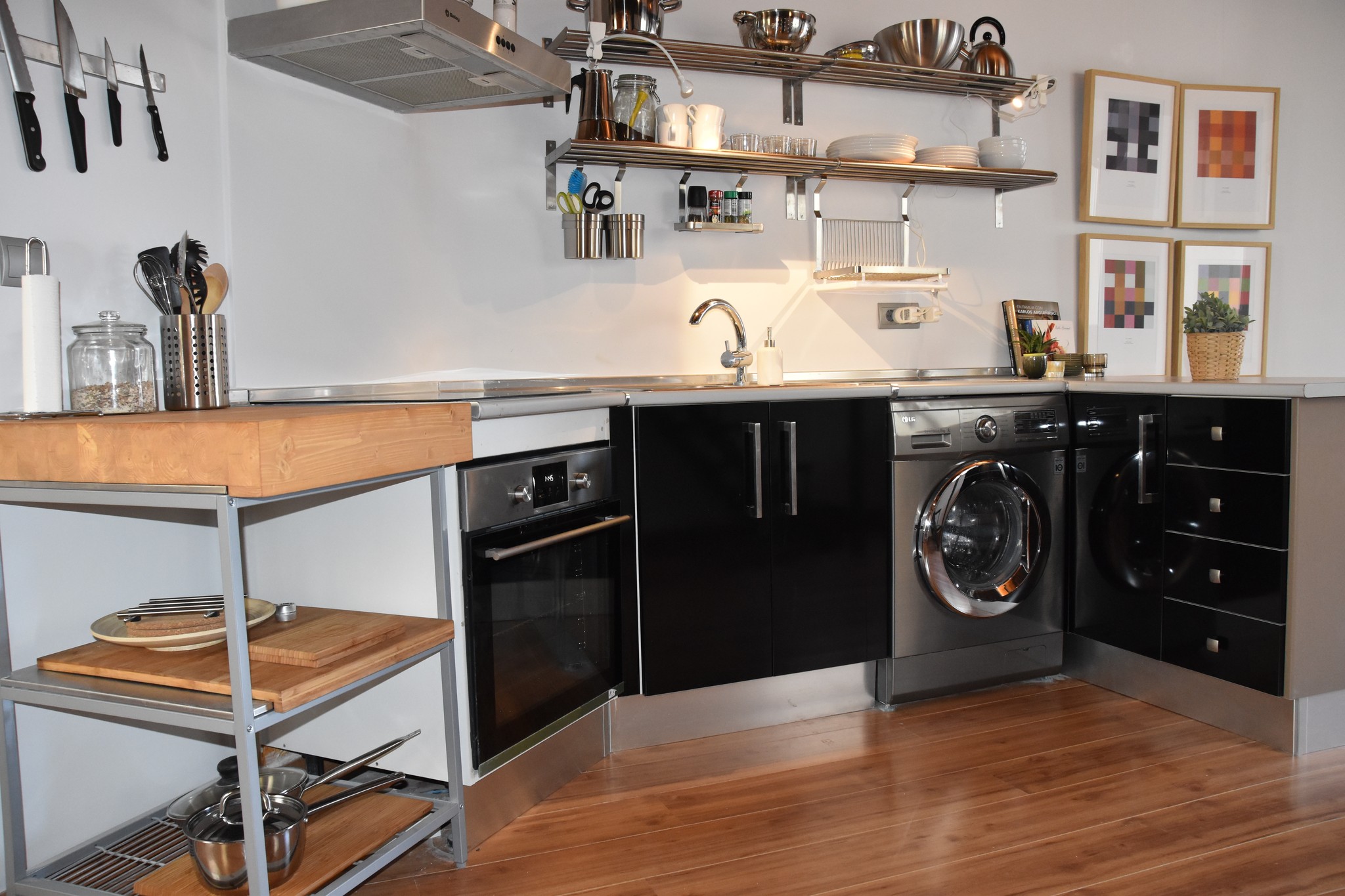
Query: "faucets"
689 298 754 383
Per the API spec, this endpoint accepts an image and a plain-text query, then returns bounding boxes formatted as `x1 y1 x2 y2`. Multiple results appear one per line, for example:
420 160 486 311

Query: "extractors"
226 0 571 114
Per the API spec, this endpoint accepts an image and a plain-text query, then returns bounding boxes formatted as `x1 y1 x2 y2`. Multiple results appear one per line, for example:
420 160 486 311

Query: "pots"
164 729 422 896
565 0 682 53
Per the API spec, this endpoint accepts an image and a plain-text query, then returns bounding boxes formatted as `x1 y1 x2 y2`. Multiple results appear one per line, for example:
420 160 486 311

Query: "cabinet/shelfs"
1065 394 1344 698
542 28 1059 192
1 406 468 896
609 398 890 698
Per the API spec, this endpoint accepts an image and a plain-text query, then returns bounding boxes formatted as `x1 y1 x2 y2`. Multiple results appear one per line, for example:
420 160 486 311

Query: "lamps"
1013 74 1057 108
586 21 694 93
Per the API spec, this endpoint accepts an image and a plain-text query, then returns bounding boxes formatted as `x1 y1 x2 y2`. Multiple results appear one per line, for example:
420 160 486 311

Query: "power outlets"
878 302 920 329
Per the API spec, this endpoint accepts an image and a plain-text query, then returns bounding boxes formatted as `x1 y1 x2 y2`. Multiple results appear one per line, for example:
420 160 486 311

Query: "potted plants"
1010 328 1057 379
1181 291 1255 381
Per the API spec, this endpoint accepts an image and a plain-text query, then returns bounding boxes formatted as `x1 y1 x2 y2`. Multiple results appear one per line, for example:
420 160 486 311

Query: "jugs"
565 68 618 153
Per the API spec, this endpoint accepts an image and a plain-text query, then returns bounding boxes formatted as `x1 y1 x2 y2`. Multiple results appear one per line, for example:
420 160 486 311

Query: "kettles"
959 16 1017 89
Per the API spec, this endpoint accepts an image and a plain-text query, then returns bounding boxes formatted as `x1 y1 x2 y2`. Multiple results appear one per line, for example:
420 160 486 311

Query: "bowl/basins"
873 19 965 81
977 136 1027 169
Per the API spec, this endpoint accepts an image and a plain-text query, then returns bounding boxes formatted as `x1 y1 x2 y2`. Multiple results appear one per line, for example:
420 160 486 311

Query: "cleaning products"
755 326 785 385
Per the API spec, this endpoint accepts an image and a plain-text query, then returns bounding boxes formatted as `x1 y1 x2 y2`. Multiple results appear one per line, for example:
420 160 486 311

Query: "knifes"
0 0 169 175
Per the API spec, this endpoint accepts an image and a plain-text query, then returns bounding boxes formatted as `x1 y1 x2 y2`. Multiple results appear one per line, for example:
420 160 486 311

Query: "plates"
1053 353 1083 376
912 145 979 167
825 133 918 163
89 598 277 652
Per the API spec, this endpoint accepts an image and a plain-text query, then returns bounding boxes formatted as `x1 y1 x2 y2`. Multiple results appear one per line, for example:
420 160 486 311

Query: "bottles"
686 186 753 224
613 74 661 143
65 310 160 416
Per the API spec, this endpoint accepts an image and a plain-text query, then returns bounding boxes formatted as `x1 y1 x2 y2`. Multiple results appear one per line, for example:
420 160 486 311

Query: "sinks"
584 385 724 392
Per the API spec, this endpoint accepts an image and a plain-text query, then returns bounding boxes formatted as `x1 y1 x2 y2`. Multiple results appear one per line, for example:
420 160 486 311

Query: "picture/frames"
1171 241 1272 377
1079 68 1181 228
1078 233 1174 379
1174 84 1281 229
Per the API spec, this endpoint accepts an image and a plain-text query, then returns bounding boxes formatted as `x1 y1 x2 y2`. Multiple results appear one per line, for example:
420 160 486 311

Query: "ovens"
457 446 632 777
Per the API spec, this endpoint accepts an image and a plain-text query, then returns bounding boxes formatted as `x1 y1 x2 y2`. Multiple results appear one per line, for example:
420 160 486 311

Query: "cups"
721 133 817 157
1081 353 1107 377
561 213 604 259
656 103 727 151
1045 361 1065 377
604 214 645 260
